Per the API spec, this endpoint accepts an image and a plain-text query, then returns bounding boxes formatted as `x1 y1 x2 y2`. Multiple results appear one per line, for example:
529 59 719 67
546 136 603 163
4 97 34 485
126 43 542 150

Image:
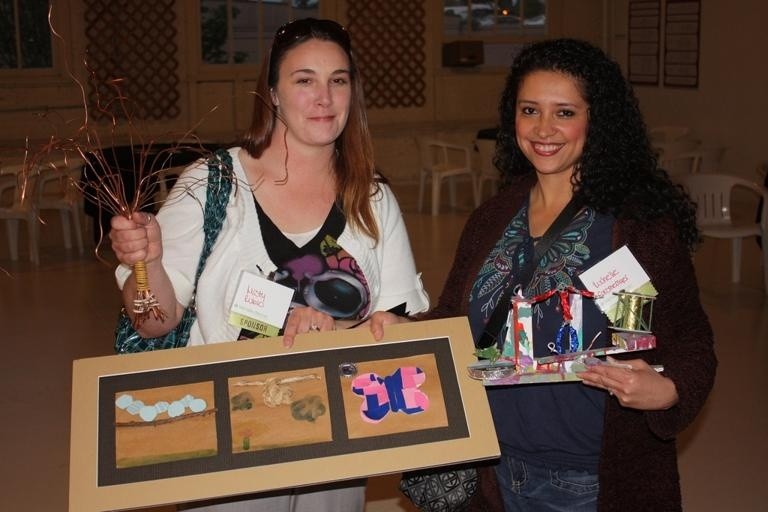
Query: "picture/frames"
69 316 499 511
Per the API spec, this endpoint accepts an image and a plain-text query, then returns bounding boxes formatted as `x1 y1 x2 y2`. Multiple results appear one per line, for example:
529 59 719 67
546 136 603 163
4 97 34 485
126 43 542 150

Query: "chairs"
414 132 483 216
474 138 505 209
17 156 90 262
684 172 768 283
0 164 47 265
656 138 701 177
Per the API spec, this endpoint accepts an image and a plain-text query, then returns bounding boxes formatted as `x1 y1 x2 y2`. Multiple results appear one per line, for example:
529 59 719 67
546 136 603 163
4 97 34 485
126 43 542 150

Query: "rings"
607 388 615 397
144 213 153 224
309 323 321 332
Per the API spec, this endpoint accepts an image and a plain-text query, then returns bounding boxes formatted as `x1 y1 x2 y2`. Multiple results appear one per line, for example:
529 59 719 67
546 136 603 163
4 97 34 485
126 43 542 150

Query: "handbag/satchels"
399 465 481 510
114 303 198 354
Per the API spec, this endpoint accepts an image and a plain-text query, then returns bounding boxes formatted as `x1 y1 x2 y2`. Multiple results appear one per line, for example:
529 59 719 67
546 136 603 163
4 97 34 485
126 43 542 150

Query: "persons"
356 36 721 512
106 13 430 511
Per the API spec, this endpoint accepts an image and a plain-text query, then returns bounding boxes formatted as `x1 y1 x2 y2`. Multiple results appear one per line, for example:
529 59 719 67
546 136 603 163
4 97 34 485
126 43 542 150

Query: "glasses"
273 17 350 50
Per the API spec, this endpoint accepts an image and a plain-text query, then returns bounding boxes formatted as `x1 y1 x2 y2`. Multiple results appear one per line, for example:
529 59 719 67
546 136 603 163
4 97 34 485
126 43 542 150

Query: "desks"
75 138 246 244
